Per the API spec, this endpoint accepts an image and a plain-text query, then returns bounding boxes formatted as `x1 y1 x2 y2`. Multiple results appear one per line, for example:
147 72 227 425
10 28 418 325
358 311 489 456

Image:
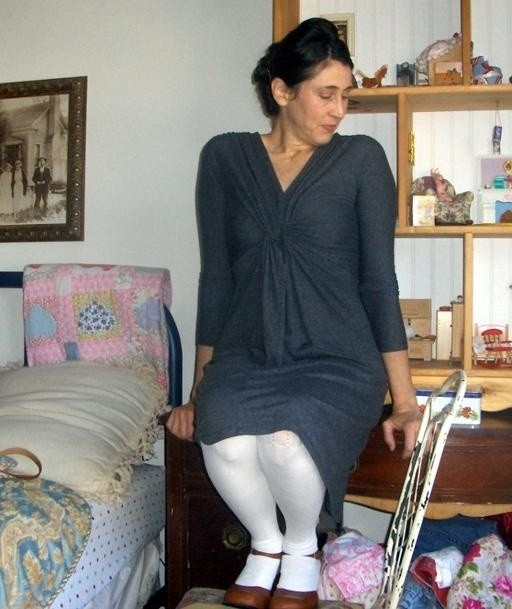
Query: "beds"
0 263 183 608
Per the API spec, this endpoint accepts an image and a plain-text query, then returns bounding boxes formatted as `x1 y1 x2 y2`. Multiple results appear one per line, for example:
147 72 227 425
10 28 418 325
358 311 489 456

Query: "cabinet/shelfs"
157 411 512 609
272 1 512 413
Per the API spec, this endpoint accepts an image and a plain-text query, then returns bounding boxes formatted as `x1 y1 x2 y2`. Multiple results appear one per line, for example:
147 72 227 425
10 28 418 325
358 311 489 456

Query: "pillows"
0 362 171 505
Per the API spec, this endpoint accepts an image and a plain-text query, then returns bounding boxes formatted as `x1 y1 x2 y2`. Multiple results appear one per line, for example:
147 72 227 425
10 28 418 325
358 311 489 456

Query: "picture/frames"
0 76 88 241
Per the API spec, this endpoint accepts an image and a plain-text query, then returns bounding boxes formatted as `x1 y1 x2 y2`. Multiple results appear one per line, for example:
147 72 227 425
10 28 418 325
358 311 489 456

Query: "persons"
164 17 426 608
33 158 51 216
0 162 12 215
13 159 27 214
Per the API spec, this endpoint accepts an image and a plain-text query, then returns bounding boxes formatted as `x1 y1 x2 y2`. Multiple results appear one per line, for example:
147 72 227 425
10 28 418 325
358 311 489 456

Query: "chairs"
176 370 468 609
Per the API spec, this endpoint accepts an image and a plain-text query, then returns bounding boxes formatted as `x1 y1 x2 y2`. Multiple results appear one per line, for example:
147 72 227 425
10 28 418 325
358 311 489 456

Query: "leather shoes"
223 549 282 608
271 547 324 609
